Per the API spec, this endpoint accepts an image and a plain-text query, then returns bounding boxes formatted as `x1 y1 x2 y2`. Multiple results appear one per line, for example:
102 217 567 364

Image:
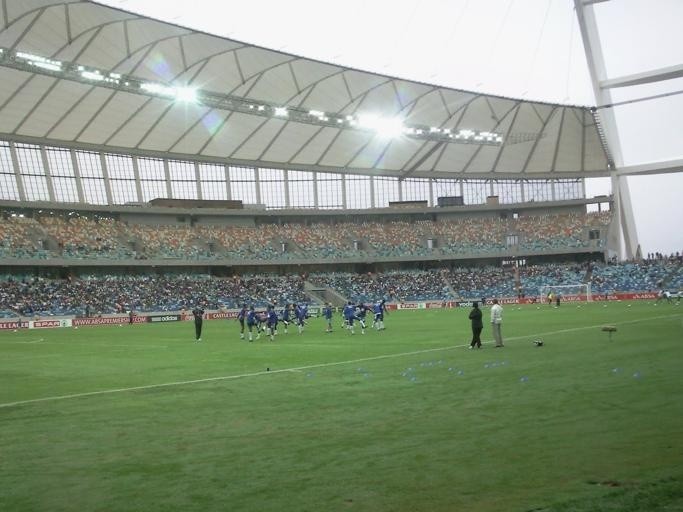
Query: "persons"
191 303 205 340
237 322 386 343
127 310 135 326
465 301 484 351
1 207 613 322
14 318 24 332
489 298 504 348
613 251 683 308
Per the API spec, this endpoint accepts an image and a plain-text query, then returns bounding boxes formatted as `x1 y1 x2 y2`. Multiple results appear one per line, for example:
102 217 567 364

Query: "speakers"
437 196 464 207
588 229 601 240
